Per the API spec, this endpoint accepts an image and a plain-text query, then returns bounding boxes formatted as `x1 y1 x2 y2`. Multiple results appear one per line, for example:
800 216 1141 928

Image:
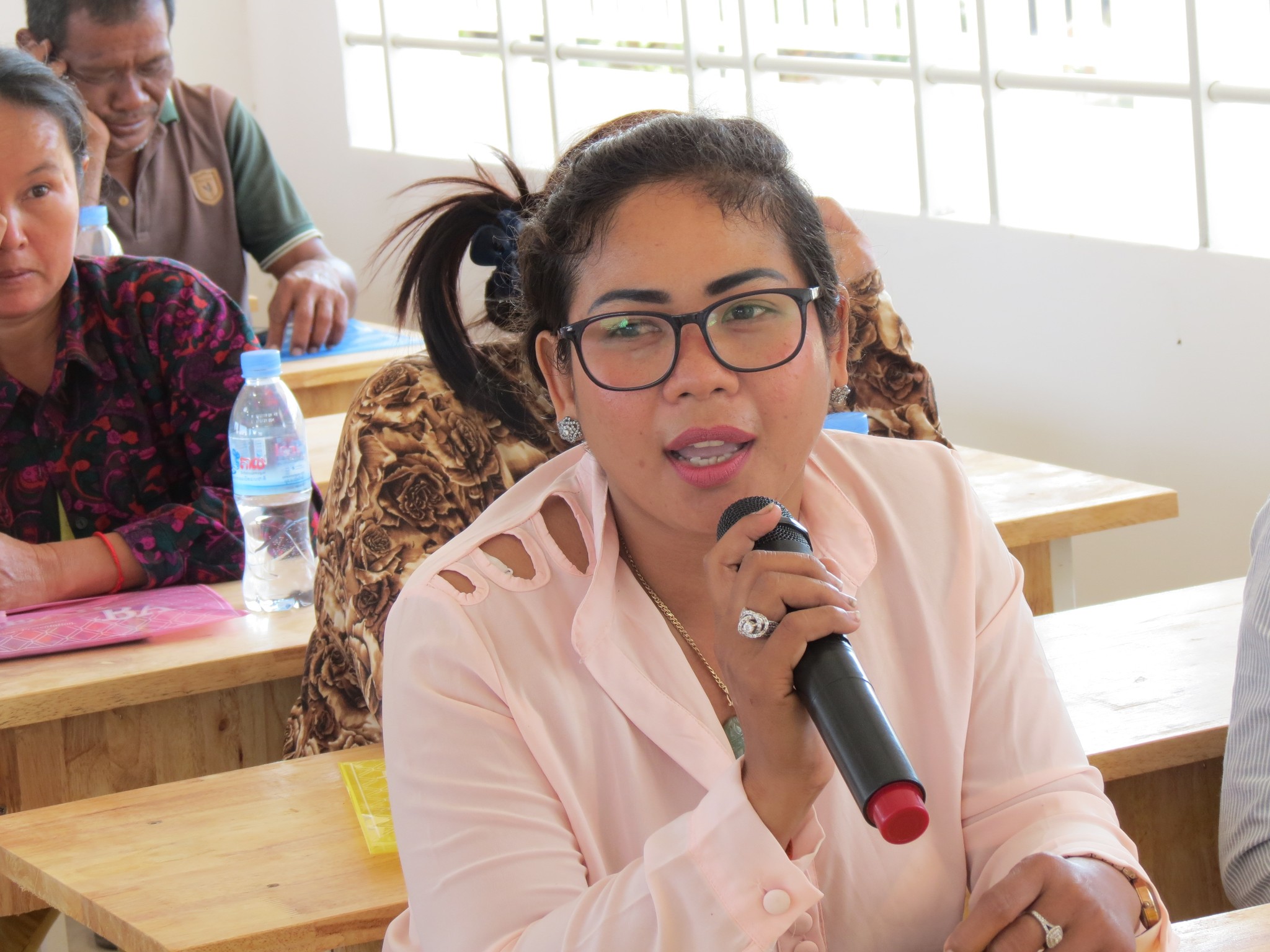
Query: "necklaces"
618 529 746 759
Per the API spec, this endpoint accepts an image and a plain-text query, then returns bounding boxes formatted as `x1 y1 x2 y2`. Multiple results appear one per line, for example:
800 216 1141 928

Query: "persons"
377 109 1175 952
16 0 357 356
283 197 958 761
0 45 323 612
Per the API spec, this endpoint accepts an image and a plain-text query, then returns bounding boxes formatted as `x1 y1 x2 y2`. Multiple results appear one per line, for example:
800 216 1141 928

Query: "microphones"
715 497 930 846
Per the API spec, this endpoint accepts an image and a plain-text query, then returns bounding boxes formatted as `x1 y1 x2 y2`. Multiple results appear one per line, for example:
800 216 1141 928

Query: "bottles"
227 350 318 614
74 204 124 257
822 412 870 437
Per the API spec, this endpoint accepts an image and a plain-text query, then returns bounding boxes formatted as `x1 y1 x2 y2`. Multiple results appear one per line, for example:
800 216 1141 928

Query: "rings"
1024 909 1063 948
738 607 778 640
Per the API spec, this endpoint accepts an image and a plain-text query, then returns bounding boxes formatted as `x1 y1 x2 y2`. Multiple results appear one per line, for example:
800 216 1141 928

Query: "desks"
1 320 1270 952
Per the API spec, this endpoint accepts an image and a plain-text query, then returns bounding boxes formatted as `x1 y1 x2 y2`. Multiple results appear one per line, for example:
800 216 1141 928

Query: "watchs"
1085 853 1159 929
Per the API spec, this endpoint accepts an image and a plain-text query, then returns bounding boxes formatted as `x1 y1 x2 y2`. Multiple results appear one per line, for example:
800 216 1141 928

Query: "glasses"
555 283 821 391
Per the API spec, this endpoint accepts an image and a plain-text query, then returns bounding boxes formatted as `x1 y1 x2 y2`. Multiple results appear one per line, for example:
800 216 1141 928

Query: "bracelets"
93 532 124 595
